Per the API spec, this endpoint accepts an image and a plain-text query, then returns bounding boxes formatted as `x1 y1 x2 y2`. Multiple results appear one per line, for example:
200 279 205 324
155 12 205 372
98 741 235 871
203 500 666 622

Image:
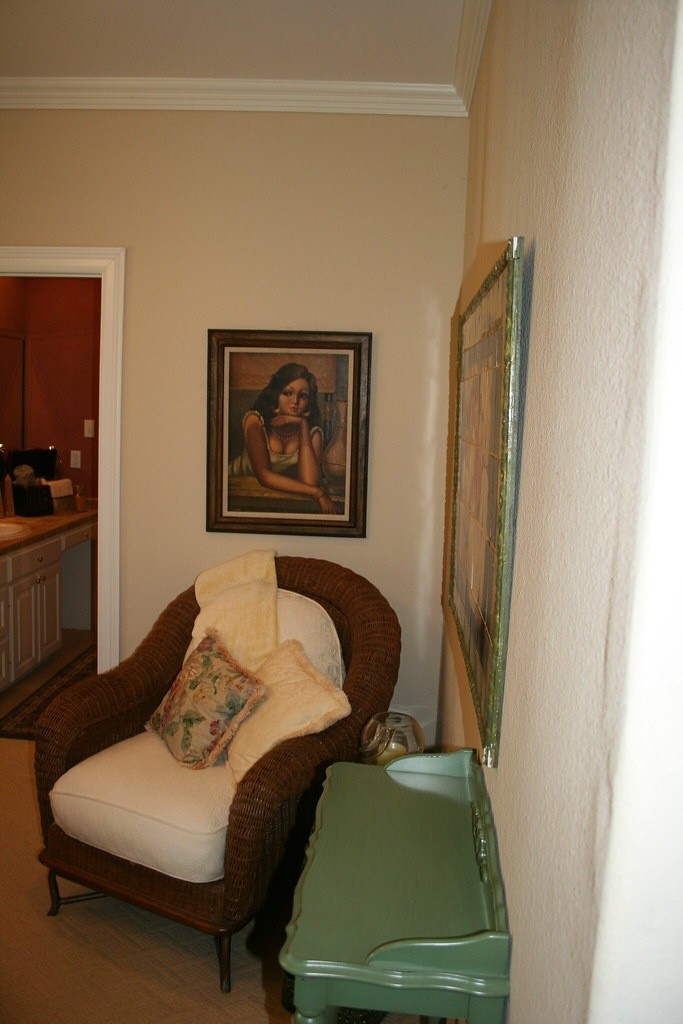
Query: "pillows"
226 638 352 783
144 627 267 770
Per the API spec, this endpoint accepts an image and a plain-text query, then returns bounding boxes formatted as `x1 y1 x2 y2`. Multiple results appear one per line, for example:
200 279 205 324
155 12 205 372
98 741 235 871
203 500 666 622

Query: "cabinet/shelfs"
0 519 97 694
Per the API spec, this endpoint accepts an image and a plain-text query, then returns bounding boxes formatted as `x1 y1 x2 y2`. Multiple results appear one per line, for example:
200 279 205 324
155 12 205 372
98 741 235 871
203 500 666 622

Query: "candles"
378 741 406 766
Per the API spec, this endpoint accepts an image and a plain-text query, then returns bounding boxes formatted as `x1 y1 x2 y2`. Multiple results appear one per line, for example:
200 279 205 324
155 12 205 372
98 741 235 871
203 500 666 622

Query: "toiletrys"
74 483 87 513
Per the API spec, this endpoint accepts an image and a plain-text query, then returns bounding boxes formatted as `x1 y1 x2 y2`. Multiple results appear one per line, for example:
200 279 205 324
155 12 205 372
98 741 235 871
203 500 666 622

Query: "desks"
280 746 513 1024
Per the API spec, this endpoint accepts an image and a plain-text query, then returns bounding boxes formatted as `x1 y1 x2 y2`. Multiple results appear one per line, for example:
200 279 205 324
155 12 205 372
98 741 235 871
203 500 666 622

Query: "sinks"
0 523 31 542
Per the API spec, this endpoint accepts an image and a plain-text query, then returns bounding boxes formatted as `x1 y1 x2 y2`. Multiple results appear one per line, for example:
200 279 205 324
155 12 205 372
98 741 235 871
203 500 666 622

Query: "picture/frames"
439 237 526 768
207 328 373 538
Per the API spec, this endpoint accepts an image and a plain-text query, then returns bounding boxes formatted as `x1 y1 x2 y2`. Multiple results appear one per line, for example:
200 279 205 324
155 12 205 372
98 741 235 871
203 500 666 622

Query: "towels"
44 479 73 499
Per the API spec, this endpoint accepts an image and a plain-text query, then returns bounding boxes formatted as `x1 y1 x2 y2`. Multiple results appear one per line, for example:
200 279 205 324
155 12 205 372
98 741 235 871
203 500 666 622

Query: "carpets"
0 644 97 741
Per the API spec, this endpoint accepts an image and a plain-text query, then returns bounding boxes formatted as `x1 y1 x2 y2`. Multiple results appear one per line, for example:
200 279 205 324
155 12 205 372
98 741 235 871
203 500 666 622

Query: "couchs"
32 555 402 994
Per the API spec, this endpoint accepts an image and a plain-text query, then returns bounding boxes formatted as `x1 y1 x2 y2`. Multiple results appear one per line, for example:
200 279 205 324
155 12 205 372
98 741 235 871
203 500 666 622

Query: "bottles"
75 484 86 511
